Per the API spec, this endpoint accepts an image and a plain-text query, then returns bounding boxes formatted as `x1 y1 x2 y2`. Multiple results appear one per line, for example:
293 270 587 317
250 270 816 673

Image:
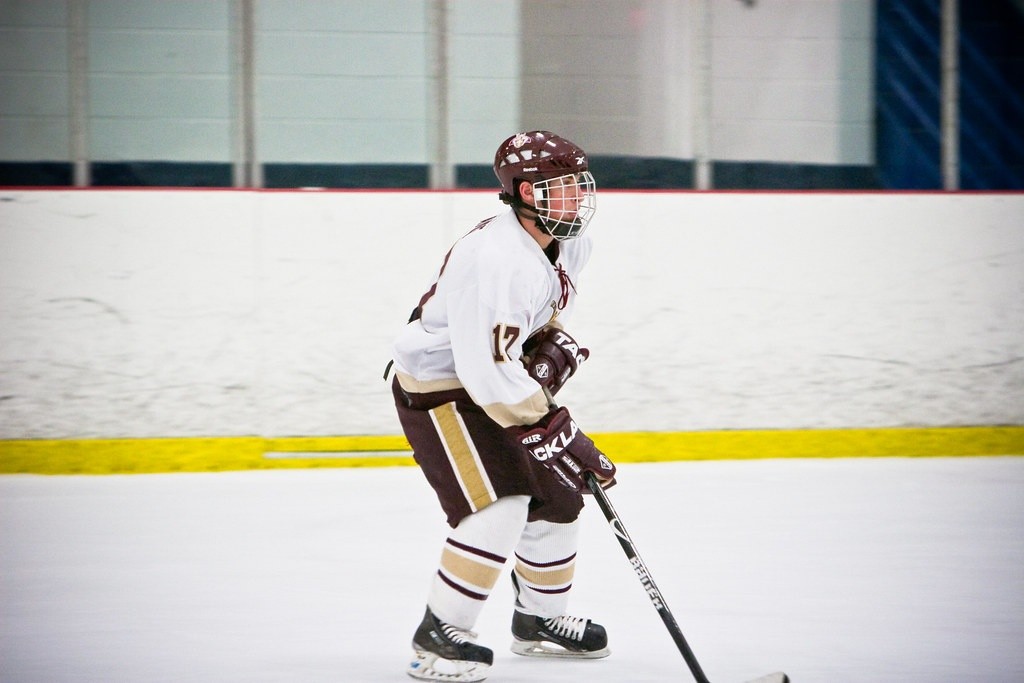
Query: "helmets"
492 130 596 240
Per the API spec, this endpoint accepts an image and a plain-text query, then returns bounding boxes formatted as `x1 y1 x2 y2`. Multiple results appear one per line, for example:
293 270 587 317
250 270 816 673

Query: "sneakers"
511 569 610 659
407 604 493 682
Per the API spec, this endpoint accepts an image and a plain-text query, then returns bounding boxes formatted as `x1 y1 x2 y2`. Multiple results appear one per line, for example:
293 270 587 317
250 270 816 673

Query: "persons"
383 130 617 683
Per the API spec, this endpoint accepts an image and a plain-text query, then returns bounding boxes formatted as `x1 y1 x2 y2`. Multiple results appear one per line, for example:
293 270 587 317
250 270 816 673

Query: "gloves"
504 407 617 500
530 328 590 389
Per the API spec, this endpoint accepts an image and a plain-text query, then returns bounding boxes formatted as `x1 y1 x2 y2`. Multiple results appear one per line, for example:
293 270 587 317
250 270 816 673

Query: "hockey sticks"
544 385 792 683
